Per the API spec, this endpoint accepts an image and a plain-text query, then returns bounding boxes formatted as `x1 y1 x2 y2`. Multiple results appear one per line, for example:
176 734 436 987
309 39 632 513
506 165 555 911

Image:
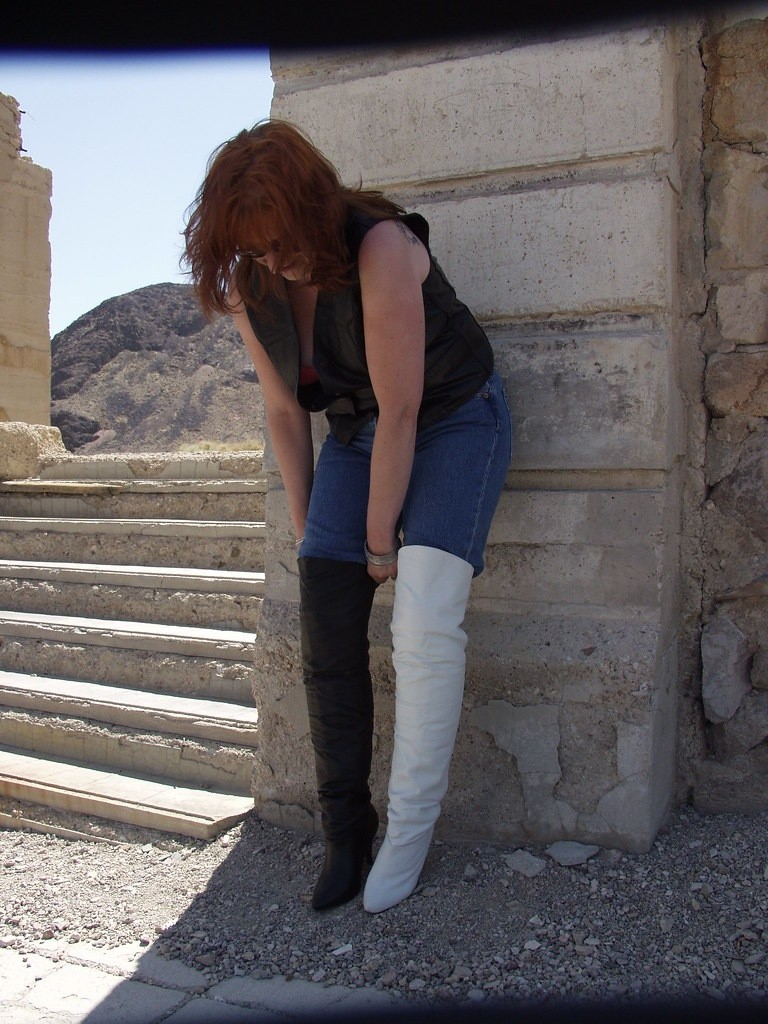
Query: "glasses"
234 232 289 258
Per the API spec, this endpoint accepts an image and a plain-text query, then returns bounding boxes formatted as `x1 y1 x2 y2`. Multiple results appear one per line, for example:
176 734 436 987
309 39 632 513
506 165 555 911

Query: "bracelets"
296 538 304 545
363 539 401 566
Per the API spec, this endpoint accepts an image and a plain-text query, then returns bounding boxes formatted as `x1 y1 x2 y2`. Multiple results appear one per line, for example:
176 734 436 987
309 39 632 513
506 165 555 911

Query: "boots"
364 546 473 914
297 557 379 910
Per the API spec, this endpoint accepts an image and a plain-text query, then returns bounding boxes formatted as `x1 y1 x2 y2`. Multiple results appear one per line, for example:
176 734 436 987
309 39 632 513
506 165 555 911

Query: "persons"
182 118 515 914
